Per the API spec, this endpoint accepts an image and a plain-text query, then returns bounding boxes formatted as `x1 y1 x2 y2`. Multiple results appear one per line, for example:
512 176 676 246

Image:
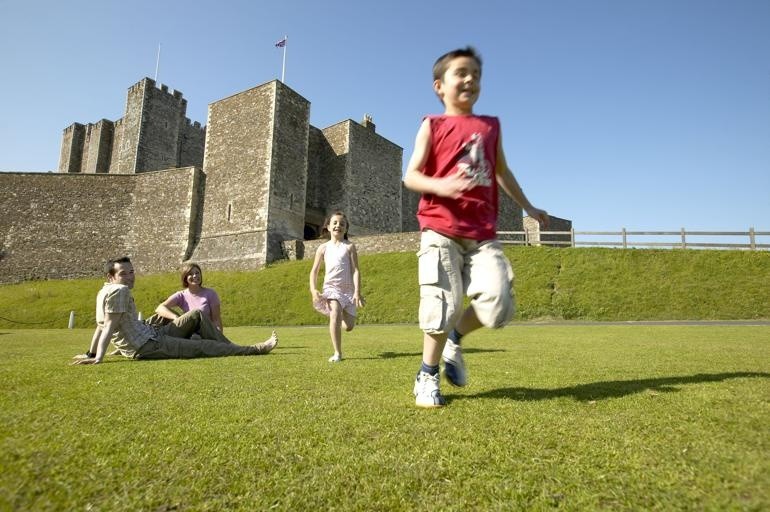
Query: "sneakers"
329 355 341 363
441 338 466 388
414 368 445 409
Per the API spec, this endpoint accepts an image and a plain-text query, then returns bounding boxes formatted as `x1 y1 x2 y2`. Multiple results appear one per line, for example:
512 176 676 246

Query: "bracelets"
85 350 96 358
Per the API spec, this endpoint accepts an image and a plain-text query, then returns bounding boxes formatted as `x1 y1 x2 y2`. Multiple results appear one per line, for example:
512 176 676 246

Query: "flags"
274 38 286 48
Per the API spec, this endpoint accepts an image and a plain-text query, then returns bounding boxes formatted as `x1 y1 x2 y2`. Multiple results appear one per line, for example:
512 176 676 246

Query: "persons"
400 47 553 408
69 256 280 367
309 210 369 362
143 262 224 339
